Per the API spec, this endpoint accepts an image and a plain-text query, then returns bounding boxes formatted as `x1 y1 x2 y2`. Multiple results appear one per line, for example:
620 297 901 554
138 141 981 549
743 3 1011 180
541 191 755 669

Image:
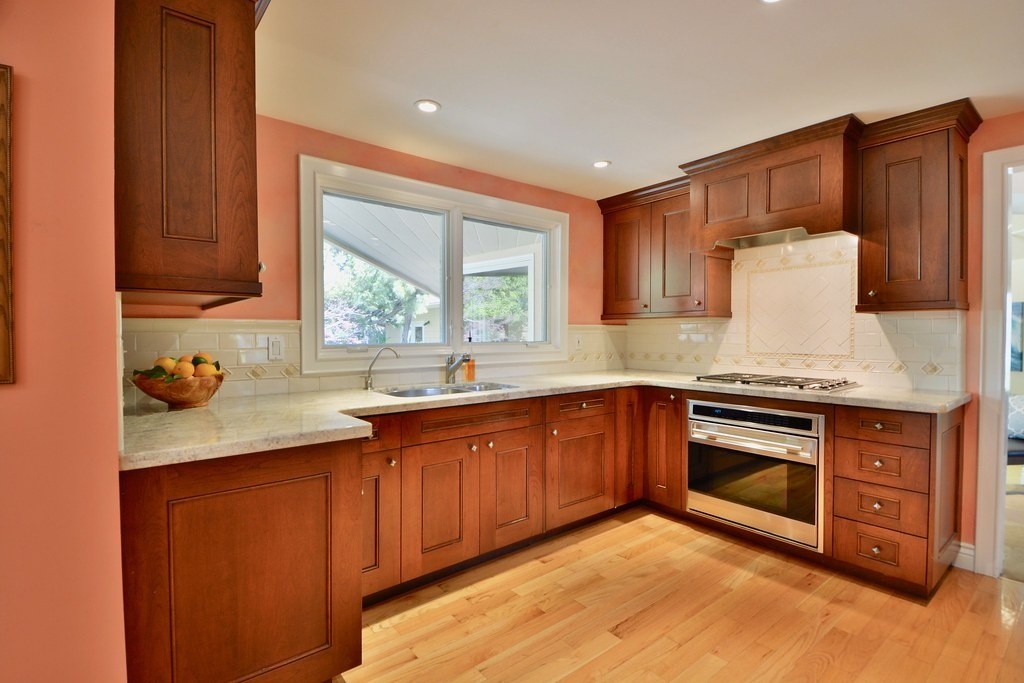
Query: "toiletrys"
462 337 476 383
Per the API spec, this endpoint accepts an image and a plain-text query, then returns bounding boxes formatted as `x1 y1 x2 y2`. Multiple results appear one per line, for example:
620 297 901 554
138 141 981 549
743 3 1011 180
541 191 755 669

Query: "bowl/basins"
134 374 226 411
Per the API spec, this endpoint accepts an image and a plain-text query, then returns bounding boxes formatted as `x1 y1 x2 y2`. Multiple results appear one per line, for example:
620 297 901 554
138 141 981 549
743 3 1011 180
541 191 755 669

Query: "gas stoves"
694 372 863 393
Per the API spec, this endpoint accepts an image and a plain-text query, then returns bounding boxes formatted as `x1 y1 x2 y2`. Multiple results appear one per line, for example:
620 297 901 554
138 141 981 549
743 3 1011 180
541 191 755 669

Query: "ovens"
684 399 824 554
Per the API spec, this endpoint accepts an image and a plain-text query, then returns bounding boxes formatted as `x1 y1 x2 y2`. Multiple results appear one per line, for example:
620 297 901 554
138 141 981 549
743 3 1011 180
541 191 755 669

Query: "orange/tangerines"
154 351 218 378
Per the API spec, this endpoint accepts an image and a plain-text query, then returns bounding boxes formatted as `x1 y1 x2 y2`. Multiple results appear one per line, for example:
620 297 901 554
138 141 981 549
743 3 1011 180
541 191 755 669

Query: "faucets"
445 351 471 384
360 347 400 390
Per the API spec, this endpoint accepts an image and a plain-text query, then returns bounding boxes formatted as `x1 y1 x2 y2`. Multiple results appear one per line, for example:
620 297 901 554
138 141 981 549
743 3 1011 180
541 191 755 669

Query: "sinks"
456 384 520 391
385 387 474 397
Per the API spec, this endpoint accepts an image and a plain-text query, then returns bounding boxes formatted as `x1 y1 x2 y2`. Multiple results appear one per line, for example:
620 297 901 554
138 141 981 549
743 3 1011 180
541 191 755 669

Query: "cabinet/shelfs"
547 389 615 533
119 414 400 682
401 396 542 581
677 114 861 252
834 404 930 588
115 0 272 317
855 97 984 312
642 388 681 511
597 174 734 319
615 387 642 507
